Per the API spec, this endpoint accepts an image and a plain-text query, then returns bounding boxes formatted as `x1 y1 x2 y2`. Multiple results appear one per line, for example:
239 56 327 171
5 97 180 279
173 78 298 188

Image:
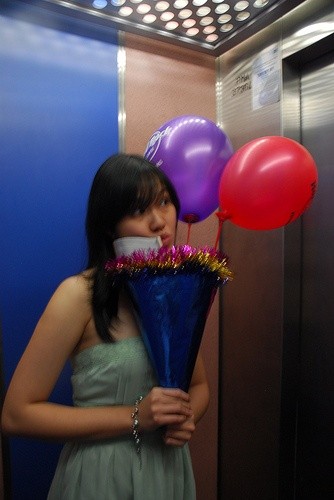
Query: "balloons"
142 116 319 254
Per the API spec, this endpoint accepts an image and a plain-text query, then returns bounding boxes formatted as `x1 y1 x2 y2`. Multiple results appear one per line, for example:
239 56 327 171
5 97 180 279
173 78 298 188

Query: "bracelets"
131 395 144 453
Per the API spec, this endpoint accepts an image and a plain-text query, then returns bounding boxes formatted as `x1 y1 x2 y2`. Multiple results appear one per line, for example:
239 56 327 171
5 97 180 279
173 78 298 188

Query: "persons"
0 154 210 500
